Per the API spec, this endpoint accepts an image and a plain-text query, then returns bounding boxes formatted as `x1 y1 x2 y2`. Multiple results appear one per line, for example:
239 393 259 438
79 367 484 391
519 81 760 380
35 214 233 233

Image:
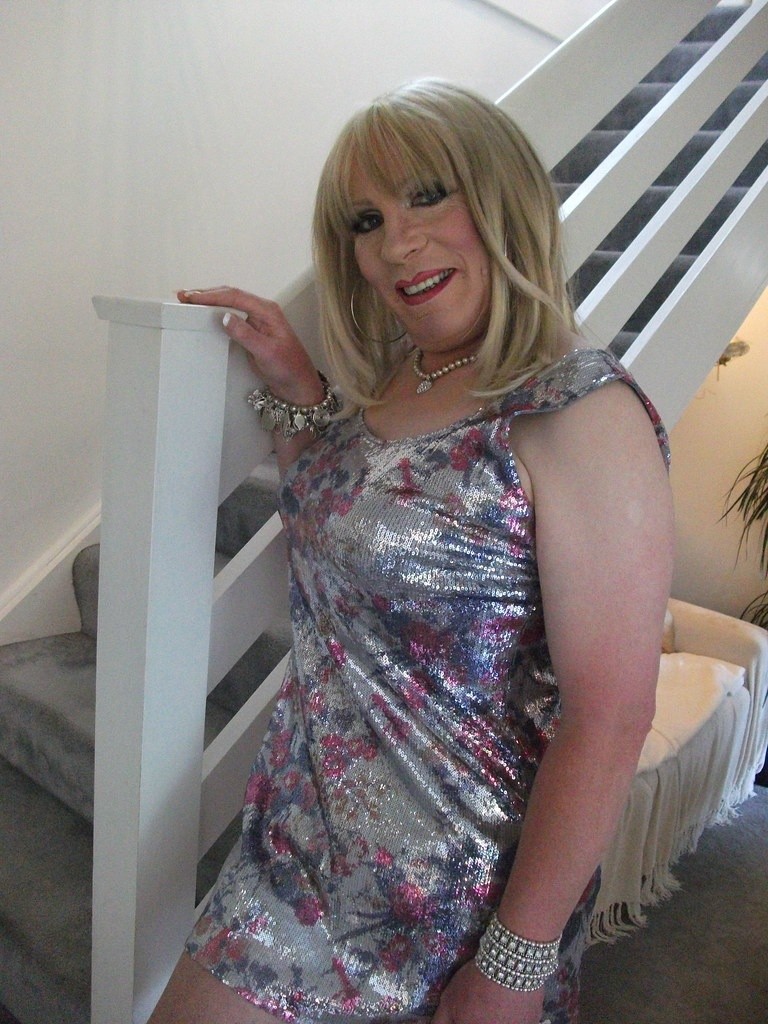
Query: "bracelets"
474 913 562 992
246 371 341 443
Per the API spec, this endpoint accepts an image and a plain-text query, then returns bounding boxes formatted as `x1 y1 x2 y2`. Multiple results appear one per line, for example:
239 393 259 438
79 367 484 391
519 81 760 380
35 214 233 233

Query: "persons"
144 78 676 1024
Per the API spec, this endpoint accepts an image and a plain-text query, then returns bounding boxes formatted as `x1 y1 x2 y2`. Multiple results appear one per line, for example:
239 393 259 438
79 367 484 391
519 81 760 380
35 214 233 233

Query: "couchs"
586 597 768 947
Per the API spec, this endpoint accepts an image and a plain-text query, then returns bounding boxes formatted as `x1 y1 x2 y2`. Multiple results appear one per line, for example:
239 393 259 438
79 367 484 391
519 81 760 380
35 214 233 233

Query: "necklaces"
412 346 479 392
